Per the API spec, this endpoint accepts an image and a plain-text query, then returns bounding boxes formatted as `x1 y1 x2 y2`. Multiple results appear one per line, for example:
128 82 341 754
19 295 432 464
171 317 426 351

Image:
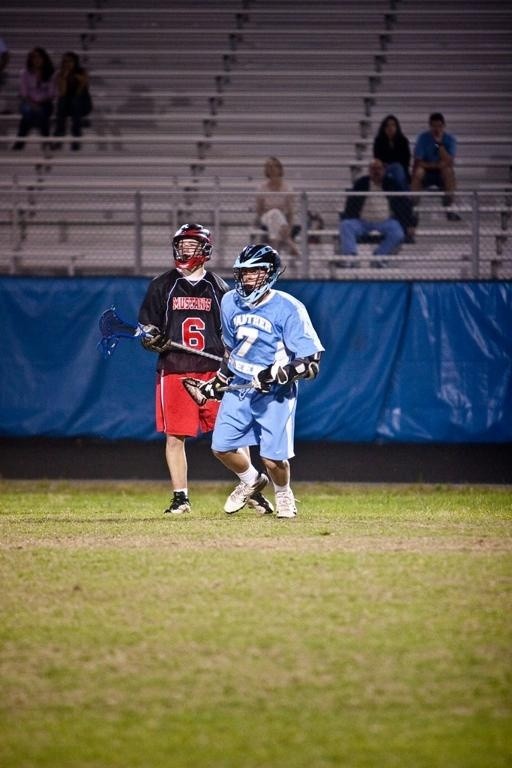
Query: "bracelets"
434 140 443 148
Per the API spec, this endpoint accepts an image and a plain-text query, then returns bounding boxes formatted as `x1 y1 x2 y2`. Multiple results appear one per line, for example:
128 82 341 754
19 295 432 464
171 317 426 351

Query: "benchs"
0 0 512 280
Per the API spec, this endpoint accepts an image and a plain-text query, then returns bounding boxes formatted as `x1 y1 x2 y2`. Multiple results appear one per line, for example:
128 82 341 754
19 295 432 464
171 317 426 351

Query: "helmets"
232 244 281 268
173 222 211 246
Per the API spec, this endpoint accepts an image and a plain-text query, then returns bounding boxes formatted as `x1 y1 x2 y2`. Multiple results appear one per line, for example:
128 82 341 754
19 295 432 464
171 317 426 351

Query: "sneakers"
223 471 269 514
163 491 191 514
248 491 274 514
274 486 297 518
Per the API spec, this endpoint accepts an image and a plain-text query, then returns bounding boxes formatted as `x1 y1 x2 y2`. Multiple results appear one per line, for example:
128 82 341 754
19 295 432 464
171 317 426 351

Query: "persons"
408 111 461 220
137 222 274 515
50 50 93 150
373 114 413 190
202 244 325 518
13 46 61 150
250 158 303 255
335 159 415 268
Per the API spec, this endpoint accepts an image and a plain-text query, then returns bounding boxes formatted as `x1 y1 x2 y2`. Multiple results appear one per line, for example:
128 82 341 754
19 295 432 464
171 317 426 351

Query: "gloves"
252 365 289 393
140 323 172 353
199 368 235 402
